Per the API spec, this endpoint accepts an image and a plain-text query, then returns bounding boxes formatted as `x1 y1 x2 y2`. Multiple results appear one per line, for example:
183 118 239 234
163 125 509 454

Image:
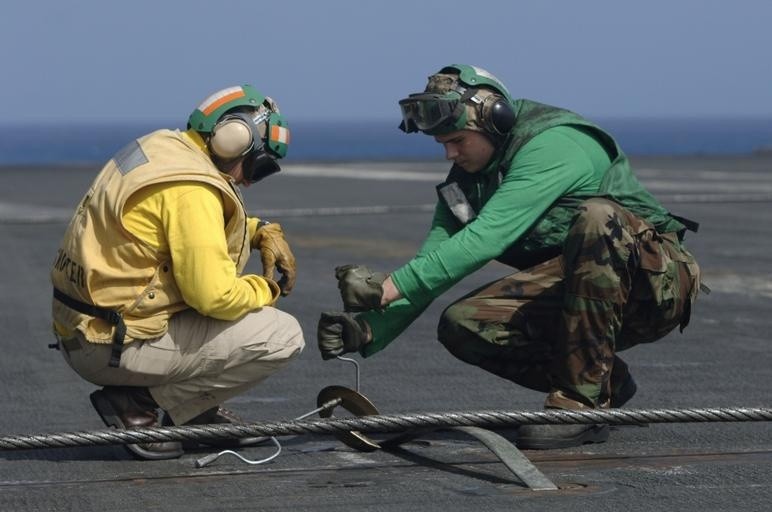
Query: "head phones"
448 81 516 136
209 110 270 161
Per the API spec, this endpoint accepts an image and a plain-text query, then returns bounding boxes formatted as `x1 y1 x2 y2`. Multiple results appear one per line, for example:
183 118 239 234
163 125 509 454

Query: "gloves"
252 221 297 296
317 312 369 360
259 276 282 307
334 263 392 313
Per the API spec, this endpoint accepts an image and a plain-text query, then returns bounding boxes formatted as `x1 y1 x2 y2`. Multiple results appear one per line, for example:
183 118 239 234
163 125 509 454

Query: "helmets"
185 82 291 160
421 62 517 139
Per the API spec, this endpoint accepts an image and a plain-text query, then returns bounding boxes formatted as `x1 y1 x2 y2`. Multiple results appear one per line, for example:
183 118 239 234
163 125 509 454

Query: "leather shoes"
161 404 276 448
515 407 610 451
607 358 637 410
90 381 186 460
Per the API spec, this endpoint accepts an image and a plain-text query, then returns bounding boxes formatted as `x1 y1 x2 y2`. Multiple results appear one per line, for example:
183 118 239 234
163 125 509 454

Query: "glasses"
398 93 472 136
245 135 282 184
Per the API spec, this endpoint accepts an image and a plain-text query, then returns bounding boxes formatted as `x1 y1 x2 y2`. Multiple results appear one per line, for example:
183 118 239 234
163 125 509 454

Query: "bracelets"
255 220 271 232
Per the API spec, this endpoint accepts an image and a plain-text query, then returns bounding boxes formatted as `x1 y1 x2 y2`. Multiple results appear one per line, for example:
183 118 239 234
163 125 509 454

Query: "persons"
46 81 306 460
318 60 711 451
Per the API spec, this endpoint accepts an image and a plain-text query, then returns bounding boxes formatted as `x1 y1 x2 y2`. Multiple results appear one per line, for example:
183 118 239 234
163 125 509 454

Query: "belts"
54 336 81 352
